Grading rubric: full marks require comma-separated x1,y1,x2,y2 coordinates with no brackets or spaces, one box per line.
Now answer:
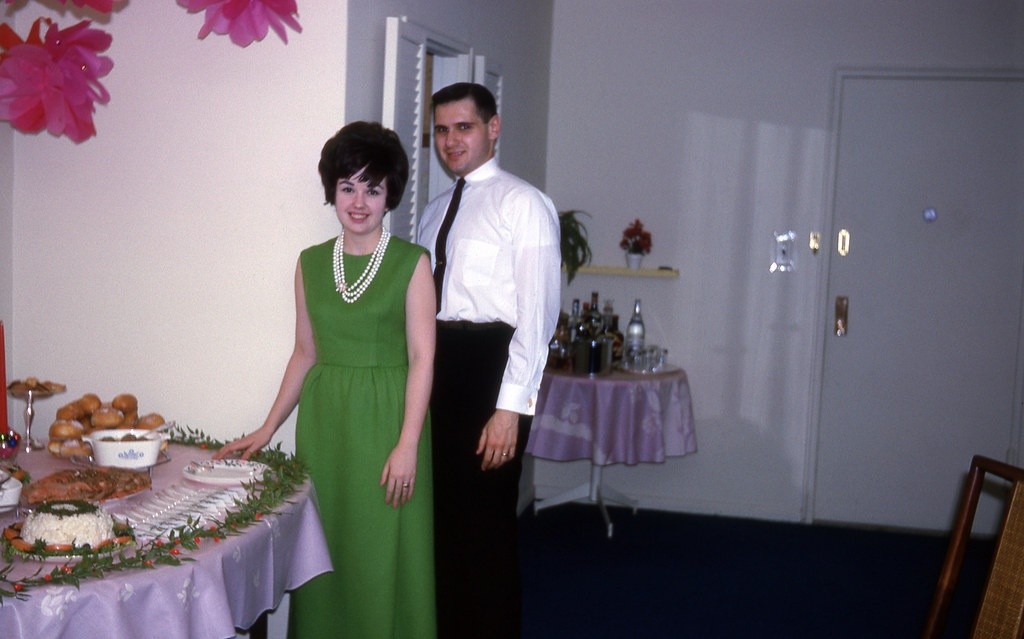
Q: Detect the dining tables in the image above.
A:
0,432,335,639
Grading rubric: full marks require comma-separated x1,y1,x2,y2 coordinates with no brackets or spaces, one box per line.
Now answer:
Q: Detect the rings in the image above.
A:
403,483,409,487
501,452,509,456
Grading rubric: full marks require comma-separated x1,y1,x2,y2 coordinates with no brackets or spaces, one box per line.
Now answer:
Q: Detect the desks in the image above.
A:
527,369,695,536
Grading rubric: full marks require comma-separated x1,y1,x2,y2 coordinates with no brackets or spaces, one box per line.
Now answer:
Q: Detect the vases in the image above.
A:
628,254,643,271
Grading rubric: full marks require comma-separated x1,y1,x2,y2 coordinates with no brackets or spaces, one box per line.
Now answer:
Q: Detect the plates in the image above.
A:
3,515,135,563
182,458,273,487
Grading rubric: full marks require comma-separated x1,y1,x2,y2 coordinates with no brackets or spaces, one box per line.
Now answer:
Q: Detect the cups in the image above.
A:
624,345,669,374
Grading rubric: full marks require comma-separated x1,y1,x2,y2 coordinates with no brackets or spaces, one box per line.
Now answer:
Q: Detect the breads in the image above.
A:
47,393,165,458
43,381,67,393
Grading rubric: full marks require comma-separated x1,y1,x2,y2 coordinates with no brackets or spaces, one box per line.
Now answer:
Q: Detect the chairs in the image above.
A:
922,454,1024,639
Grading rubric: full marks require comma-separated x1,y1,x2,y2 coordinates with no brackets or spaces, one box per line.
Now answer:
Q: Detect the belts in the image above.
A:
438,320,512,330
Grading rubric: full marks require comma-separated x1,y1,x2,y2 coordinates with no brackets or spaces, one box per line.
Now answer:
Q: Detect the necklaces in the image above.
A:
333,223,392,303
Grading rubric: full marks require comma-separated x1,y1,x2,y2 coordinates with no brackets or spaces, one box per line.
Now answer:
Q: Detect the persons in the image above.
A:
411,81,561,639
214,122,438,639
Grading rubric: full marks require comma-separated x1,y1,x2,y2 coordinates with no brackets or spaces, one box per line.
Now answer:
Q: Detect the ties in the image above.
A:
433,178,466,316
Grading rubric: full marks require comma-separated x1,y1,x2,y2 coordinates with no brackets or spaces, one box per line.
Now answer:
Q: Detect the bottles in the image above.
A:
548,292,645,380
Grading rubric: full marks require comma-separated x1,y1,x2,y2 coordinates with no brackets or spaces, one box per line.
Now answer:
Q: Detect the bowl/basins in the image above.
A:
0,472,23,512
83,428,171,468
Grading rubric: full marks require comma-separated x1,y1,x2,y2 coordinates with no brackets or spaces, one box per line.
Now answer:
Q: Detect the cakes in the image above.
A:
5,498,133,553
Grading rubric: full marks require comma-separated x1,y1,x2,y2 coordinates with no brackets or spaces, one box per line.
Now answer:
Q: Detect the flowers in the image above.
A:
176,0,303,46
0,0,117,140
619,218,653,254
